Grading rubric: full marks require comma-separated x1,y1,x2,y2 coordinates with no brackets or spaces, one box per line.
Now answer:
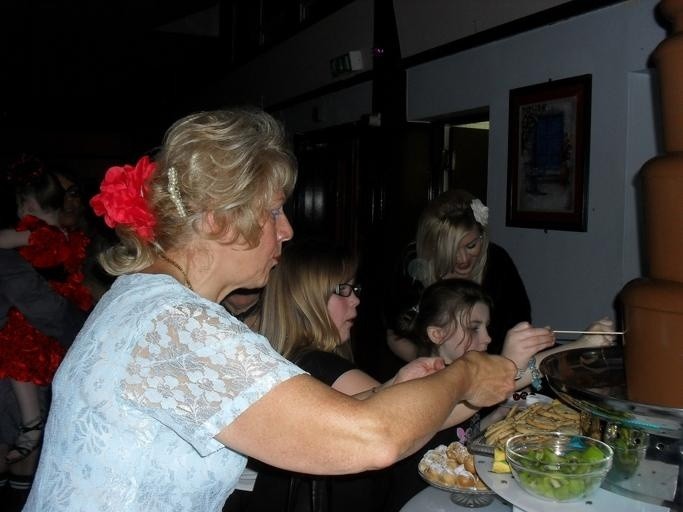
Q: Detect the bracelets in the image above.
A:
527,356,542,392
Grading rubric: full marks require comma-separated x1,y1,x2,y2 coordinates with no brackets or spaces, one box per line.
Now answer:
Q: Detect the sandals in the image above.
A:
4,418,45,466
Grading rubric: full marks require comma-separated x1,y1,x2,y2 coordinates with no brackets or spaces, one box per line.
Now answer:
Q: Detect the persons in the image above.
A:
48,162,106,308
414,278,617,491
386,188,532,443
253,243,555,512
19,104,521,512
0,244,90,511
0,172,91,464
217,288,289,511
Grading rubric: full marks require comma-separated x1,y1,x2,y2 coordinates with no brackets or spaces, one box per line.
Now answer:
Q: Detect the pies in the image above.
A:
484,398,581,448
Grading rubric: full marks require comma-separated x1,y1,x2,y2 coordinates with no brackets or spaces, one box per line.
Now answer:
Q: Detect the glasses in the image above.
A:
330,282,363,298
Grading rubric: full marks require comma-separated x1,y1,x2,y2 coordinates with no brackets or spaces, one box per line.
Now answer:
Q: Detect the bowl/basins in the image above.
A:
605,425,650,486
504,430,615,503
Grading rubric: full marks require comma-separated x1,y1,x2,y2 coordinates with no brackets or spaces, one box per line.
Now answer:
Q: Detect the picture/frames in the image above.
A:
503,73,592,232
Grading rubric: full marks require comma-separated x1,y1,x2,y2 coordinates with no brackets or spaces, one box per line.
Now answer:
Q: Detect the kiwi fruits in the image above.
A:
519,445,606,501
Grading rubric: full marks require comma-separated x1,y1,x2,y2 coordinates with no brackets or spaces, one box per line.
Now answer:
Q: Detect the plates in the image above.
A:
497,390,554,410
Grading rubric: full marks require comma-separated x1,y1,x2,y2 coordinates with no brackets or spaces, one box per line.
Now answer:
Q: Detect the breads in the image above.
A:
418,441,488,491
